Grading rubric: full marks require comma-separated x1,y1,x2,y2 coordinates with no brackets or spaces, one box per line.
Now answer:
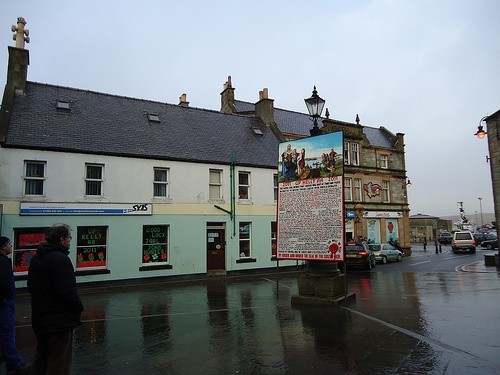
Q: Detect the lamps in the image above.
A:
406,180,412,187
474,116,488,139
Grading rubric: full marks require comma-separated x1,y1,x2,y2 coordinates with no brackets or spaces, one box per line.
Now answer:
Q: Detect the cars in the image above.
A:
368,243,404,264
345,241,376,270
439,229,498,254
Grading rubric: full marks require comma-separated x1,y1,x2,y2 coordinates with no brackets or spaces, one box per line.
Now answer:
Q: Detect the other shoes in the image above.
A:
6,363,31,375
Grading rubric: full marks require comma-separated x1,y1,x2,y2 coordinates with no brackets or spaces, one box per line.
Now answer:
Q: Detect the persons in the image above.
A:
27,222,85,375
0,236,34,375
347,235,427,254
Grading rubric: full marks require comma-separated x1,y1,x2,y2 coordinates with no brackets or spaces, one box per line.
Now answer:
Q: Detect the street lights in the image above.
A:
290,85,356,310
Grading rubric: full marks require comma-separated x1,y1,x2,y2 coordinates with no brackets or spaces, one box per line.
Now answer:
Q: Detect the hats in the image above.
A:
0,236,10,250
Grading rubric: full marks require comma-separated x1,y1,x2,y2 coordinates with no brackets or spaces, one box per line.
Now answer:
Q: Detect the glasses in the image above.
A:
64,236,72,241
